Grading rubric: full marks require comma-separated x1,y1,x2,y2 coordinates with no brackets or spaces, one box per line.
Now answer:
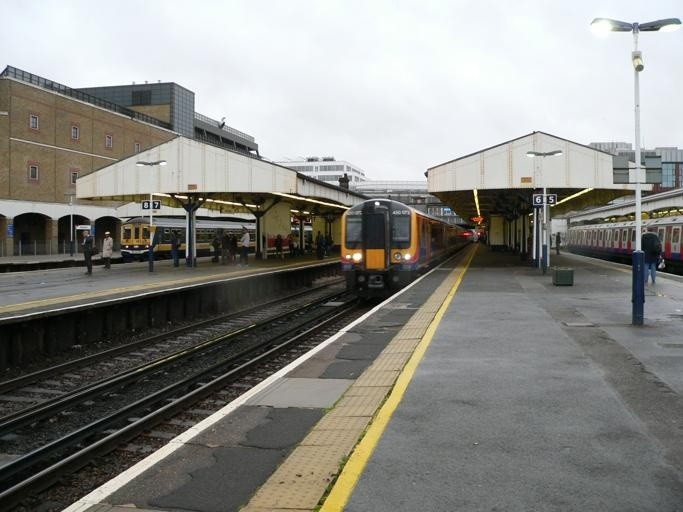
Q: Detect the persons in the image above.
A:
80,230,93,274
212,227,251,267
315,231,322,249
556,231,562,255
275,234,284,259
641,226,662,284
102,231,114,269
287,233,295,256
169,230,182,267
324,232,332,253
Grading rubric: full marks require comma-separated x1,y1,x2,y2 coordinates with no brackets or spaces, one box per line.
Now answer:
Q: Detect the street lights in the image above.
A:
589,17,683,326
526,150,564,276
136,160,167,272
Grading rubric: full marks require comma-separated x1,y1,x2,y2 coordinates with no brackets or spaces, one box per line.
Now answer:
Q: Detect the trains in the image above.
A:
566,188,683,276
120,217,313,263
340,198,473,301
